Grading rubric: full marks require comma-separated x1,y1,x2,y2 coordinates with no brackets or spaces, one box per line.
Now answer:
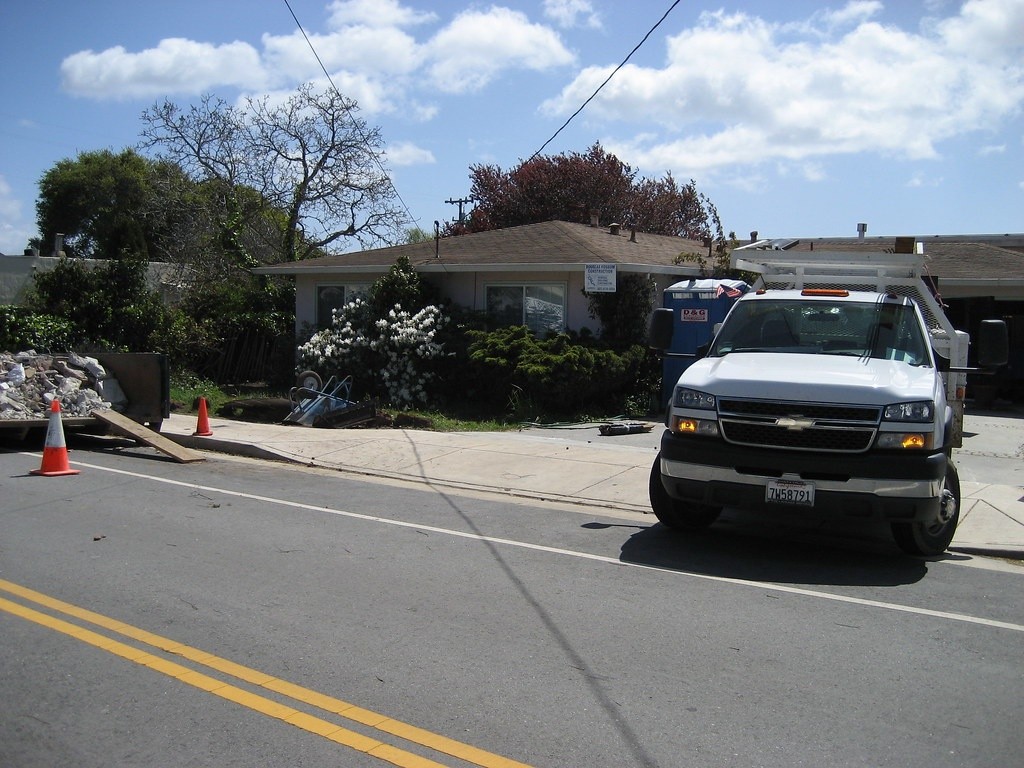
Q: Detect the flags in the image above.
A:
717,284,742,298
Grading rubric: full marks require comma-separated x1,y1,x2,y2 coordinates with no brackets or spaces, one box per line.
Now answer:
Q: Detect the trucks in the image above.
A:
648,238,1009,558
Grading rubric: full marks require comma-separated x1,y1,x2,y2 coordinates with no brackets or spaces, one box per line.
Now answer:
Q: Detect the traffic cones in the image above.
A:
29,399,81,477
191,396,213,436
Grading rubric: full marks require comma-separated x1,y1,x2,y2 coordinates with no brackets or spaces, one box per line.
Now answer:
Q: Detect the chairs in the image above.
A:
867,319,897,350
762,320,796,346
